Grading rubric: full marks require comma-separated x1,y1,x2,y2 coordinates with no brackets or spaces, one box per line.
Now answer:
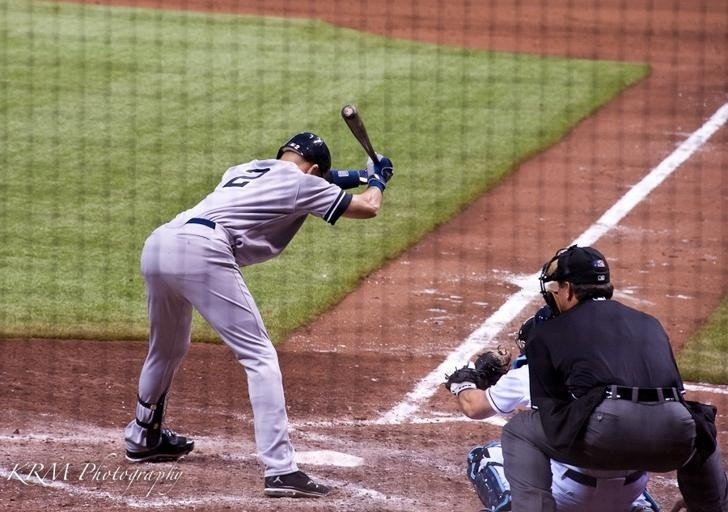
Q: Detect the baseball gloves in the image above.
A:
471,348,512,388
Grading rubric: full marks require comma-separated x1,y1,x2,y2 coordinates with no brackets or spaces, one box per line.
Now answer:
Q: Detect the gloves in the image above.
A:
366,152,394,193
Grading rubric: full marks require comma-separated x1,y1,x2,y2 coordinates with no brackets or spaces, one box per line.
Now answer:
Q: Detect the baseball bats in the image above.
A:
342,104,380,168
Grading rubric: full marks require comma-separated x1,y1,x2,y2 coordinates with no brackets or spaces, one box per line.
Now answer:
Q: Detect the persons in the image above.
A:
443,259,660,510
122,132,392,496
500,245,727,509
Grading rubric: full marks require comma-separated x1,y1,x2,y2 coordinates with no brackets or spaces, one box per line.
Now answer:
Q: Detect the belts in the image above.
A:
603,384,685,403
185,216,218,231
563,467,646,489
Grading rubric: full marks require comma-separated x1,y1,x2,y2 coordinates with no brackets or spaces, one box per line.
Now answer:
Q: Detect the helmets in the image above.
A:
538,243,611,316
276,131,332,176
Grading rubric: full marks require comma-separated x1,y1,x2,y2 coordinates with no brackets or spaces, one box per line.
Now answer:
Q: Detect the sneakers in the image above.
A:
125,431,196,463
262,469,331,498
